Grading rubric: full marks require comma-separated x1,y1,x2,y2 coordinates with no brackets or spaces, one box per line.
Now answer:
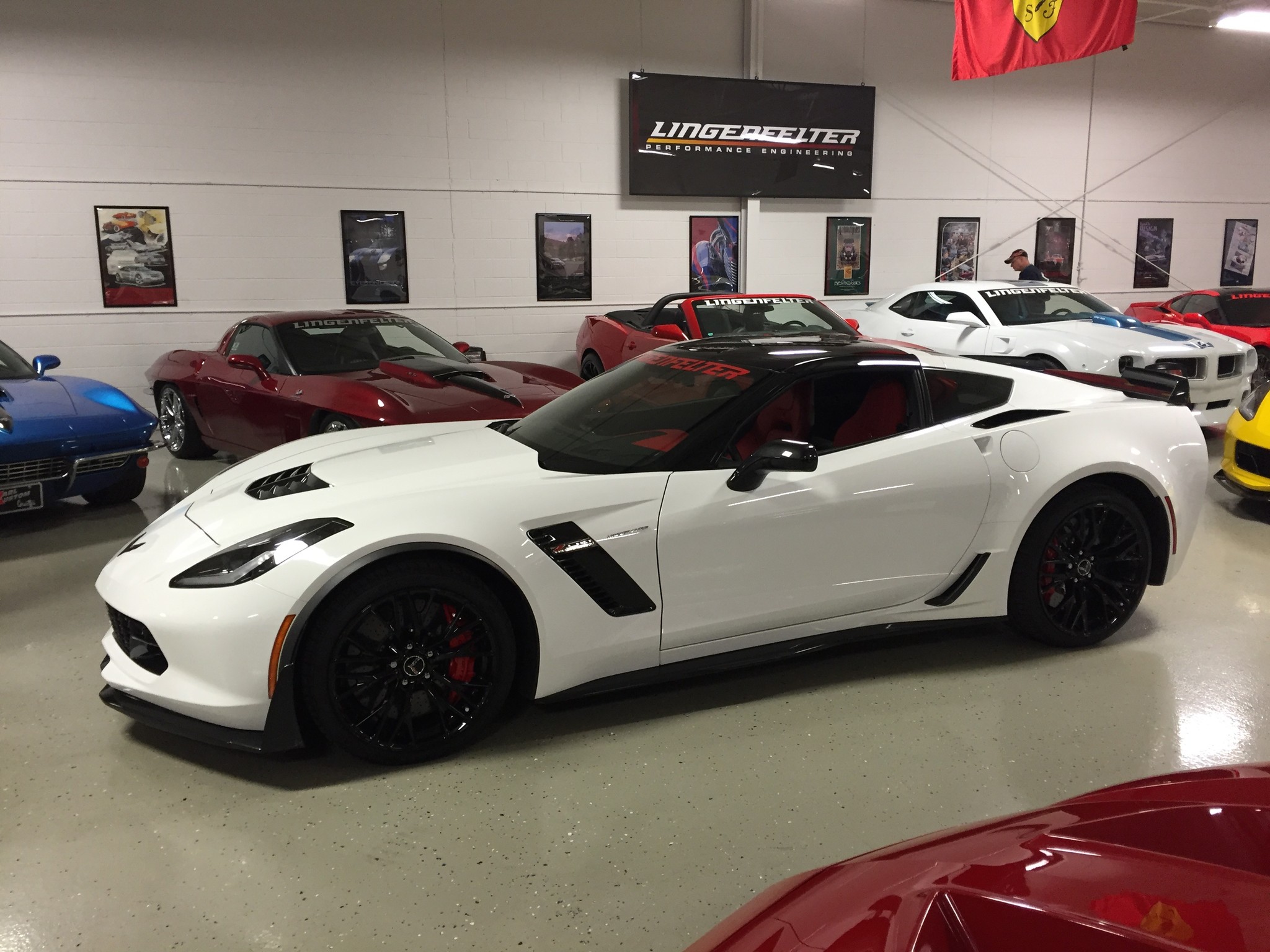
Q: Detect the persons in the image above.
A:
1004,249,1053,314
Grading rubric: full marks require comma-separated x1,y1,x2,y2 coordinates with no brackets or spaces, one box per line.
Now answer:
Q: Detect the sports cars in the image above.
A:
104,240,169,288
576,291,935,383
685,763,1270,951
841,278,1269,500
92,334,1211,766
0,339,167,518
547,257,566,269
145,308,613,462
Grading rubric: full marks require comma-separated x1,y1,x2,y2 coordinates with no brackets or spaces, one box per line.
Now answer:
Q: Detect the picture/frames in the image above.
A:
1220,219,1259,287
93,205,178,308
689,215,739,293
535,212,592,302
824,216,872,296
1133,218,1174,288
1034,217,1076,284
340,209,410,305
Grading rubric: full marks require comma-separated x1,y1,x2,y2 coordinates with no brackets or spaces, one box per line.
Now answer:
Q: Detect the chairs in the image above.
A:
289,328,328,372
331,323,398,365
832,378,906,450
736,381,814,458
742,304,766,332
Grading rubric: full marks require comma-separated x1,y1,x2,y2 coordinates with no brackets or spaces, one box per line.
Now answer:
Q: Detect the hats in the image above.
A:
1004,249,1028,264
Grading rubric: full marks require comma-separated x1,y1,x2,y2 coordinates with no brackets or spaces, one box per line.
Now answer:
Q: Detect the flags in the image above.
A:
950,1,1139,81
